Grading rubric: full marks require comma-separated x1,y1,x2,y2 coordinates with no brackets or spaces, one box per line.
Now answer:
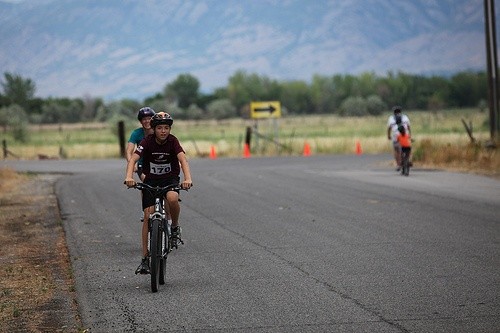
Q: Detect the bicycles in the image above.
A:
124,170,193,292
400,150,410,175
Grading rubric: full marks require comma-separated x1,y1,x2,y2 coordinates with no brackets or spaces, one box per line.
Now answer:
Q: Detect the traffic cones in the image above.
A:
303,142,311,157
356,142,362,155
209,145,217,159
243,143,252,158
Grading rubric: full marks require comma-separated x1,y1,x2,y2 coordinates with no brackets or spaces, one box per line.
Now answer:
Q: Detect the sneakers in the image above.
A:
141,257,151,274
169,224,182,246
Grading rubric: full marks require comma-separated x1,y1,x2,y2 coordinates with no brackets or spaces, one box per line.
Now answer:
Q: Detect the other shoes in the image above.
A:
140,212,144,220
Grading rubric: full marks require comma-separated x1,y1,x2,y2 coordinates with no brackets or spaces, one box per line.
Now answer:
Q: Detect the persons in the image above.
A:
126,107,157,221
388,107,412,176
125,112,193,274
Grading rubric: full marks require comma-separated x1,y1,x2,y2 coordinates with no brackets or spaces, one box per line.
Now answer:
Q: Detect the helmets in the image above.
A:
149,111,174,127
138,107,156,120
